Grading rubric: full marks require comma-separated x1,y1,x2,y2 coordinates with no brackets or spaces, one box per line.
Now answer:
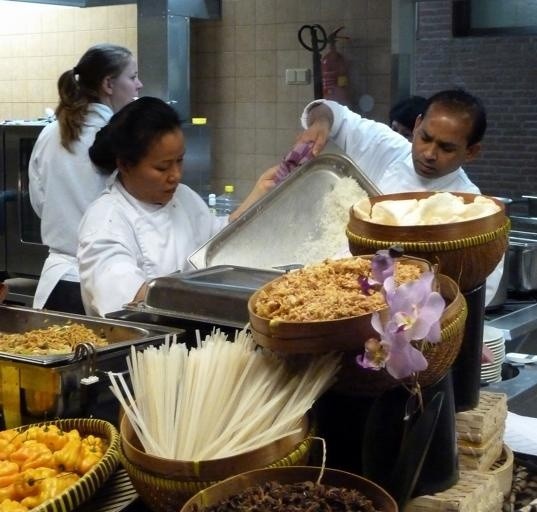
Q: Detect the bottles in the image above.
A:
209,194,217,216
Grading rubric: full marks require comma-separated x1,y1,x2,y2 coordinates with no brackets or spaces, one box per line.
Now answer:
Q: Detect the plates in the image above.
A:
480,324,507,385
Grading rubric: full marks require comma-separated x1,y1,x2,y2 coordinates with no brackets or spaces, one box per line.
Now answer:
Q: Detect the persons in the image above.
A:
77,96,280,320
293,90,505,306
389,95,427,142
28,43,144,315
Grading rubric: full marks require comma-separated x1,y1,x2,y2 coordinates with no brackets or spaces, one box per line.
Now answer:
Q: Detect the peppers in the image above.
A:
0,425,103,511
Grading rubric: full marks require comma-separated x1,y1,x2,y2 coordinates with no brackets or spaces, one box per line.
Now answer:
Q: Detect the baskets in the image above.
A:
406,273,470,390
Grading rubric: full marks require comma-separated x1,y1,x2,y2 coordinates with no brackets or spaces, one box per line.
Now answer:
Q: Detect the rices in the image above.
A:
299,175,371,266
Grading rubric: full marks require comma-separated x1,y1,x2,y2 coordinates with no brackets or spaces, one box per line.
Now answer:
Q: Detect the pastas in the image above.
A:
107,321,344,461
